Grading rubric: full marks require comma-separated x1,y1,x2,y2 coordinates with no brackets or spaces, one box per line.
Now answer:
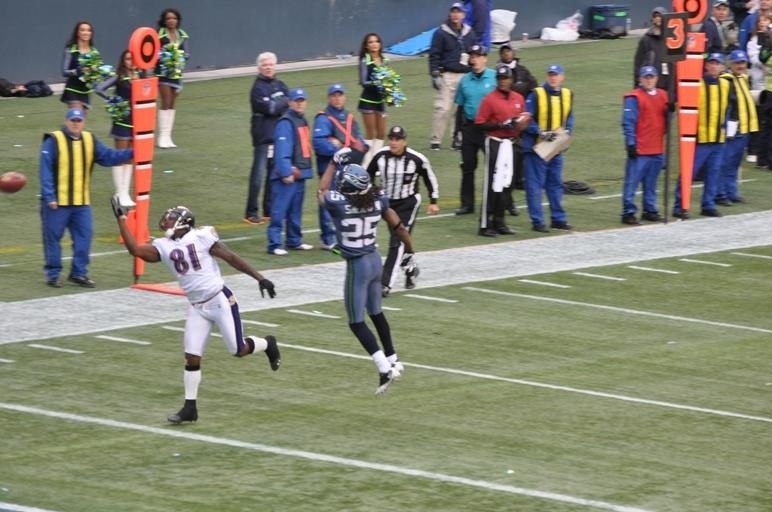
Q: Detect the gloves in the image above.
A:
627,146,636,159
451,131,463,147
332,147,352,165
431,71,442,90
400,253,416,273
110,196,130,219
540,130,557,142
498,118,516,129
256,279,276,299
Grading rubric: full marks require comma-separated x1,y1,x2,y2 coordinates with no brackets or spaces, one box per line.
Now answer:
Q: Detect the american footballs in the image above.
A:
0,172,29,193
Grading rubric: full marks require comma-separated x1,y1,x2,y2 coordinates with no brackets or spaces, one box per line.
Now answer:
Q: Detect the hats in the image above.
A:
547,65,564,75
712,0,729,8
639,65,657,77
327,84,345,94
387,125,408,139
499,43,512,52
494,65,513,78
652,6,668,15
467,44,488,56
65,109,84,120
449,3,464,11
288,89,306,100
729,50,748,64
706,52,726,64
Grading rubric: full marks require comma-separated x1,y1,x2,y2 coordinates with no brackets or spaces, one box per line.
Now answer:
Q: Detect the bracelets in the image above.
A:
393,220,402,231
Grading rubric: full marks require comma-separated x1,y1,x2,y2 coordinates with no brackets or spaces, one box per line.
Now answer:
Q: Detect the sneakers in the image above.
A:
67,274,96,288
496,226,516,235
243,216,266,225
622,215,638,225
641,212,663,221
406,267,420,288
673,210,689,219
290,243,315,250
375,365,400,396
320,242,335,250
478,228,496,237
508,203,520,216
701,208,722,217
381,286,390,297
264,335,281,371
391,359,404,373
532,225,549,233
551,223,573,230
716,198,733,206
428,144,440,151
733,197,745,204
755,160,768,169
262,216,271,221
450,145,461,152
268,248,289,256
168,408,199,424
48,280,63,288
515,177,526,189
455,206,474,215
746,155,757,163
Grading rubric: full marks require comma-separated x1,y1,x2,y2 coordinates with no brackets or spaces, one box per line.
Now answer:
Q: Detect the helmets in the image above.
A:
335,163,370,194
159,206,194,230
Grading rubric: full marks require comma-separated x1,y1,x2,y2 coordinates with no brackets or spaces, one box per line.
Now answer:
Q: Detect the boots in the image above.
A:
155,109,169,149
168,109,176,148
362,139,373,169
124,164,136,206
110,165,129,207
373,138,384,157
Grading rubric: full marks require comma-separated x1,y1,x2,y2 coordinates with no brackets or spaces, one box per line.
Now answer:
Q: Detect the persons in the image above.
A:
38,107,133,288
474,65,515,237
311,83,364,251
495,44,537,190
155,8,191,147
522,63,573,233
429,2,478,152
621,65,671,226
453,44,519,217
465,0,492,53
357,33,395,141
633,7,676,109
243,52,289,225
317,147,404,397
110,195,280,425
267,88,313,256
59,21,102,114
367,125,440,298
94,50,140,209
672,0,771,219
